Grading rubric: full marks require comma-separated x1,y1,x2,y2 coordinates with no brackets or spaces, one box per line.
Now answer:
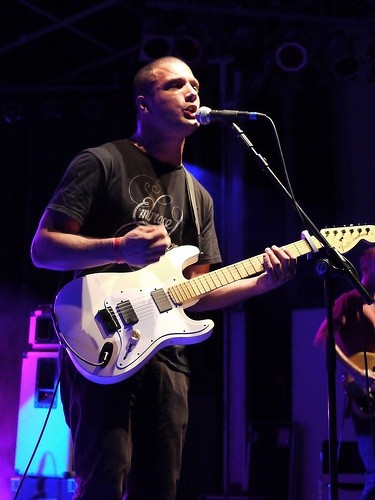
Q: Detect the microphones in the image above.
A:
196,106,266,125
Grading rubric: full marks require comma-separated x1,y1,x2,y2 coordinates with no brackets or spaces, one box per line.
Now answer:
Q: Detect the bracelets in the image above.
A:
113,237,120,264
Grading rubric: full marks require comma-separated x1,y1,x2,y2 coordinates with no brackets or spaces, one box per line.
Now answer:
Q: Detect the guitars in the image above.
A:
343,350,375,422
52,222,375,388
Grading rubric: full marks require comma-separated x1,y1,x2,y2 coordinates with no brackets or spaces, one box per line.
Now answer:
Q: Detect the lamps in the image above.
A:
4,98,102,123
327,25,360,79
140,12,208,65
276,26,309,72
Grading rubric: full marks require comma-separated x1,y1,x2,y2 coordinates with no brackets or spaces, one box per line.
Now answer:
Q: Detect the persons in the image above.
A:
31,57,293,500
